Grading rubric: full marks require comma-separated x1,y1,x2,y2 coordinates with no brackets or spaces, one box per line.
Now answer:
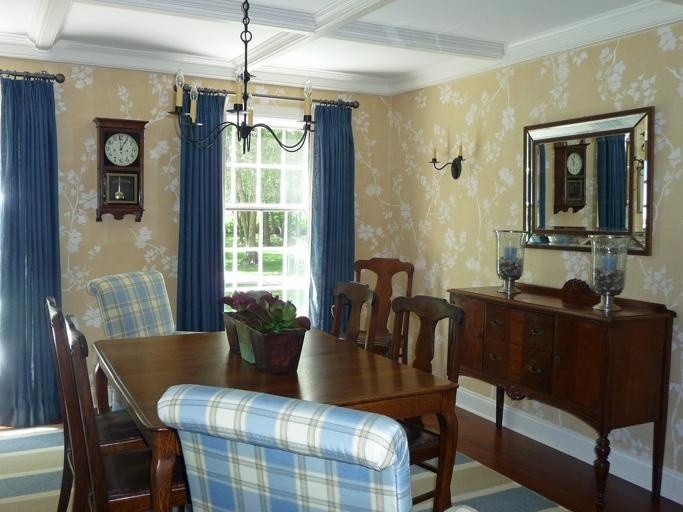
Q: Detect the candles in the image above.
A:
502,246,518,260
601,254,616,271
458,145,462,157
432,148,436,157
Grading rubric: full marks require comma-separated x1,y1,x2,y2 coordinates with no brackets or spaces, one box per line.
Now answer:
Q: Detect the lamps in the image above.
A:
167,0,315,158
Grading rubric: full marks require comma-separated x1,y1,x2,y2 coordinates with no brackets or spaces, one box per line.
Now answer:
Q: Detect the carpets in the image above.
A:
0,423,570,512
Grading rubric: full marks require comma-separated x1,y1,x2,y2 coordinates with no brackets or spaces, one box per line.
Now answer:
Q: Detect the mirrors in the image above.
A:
518,106,654,256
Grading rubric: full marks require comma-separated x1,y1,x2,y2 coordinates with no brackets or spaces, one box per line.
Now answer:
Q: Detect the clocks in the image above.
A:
93,114,148,224
552,142,587,214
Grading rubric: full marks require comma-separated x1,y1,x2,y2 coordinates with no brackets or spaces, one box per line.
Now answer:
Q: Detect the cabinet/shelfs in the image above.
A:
444,285,677,511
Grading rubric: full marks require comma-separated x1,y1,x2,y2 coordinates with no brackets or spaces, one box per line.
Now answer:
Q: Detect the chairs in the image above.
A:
43,258,464,512
